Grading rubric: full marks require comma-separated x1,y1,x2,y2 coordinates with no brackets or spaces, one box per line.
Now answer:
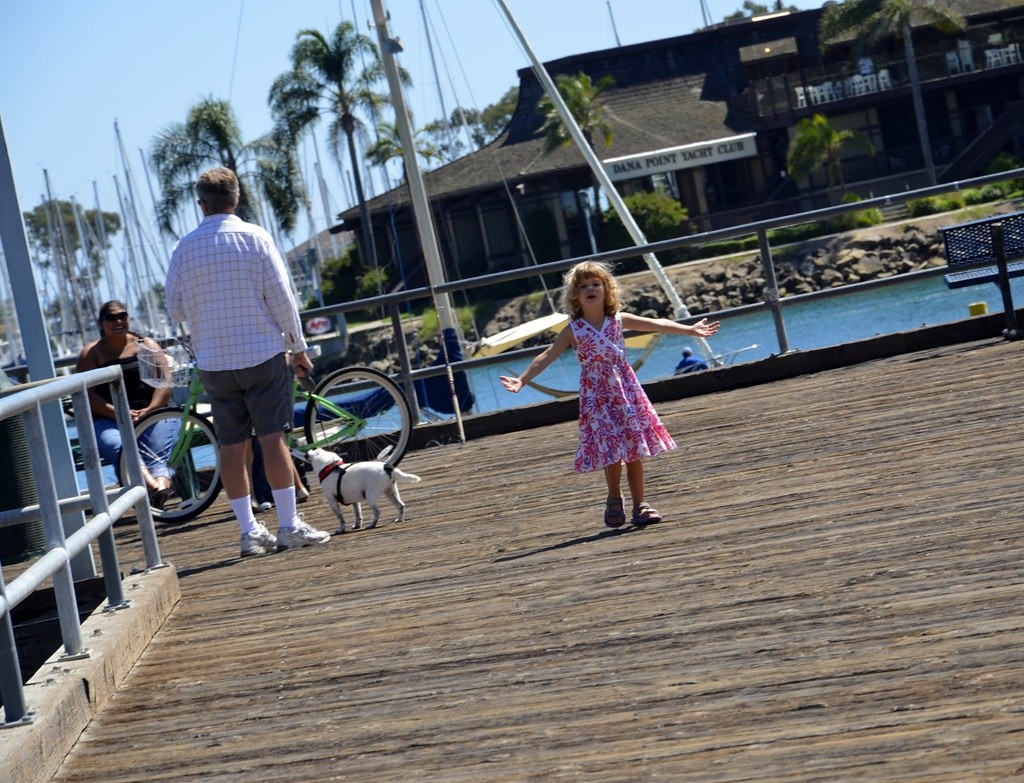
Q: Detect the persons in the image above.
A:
163,166,331,560
675,347,708,375
499,261,721,529
72,299,176,506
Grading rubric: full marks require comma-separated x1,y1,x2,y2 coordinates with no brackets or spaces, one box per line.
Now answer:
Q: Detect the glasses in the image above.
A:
102,311,128,322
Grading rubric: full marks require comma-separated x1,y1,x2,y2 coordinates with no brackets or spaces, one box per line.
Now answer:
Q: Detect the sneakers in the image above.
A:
240,520,278,557
277,512,331,548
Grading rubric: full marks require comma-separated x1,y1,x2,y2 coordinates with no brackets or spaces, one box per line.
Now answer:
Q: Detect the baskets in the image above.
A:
135,336,192,388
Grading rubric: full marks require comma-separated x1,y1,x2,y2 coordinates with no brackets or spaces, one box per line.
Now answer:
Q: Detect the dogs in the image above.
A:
308,447,422,534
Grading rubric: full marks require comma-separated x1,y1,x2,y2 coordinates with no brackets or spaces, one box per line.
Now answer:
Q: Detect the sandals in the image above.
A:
630,502,661,524
605,497,625,527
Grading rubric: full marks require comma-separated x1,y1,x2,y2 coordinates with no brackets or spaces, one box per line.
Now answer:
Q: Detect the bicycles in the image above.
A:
116,319,414,526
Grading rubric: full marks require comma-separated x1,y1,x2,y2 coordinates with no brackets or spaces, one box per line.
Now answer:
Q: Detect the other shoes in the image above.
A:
251,500,265,513
150,488,175,509
295,487,309,504
